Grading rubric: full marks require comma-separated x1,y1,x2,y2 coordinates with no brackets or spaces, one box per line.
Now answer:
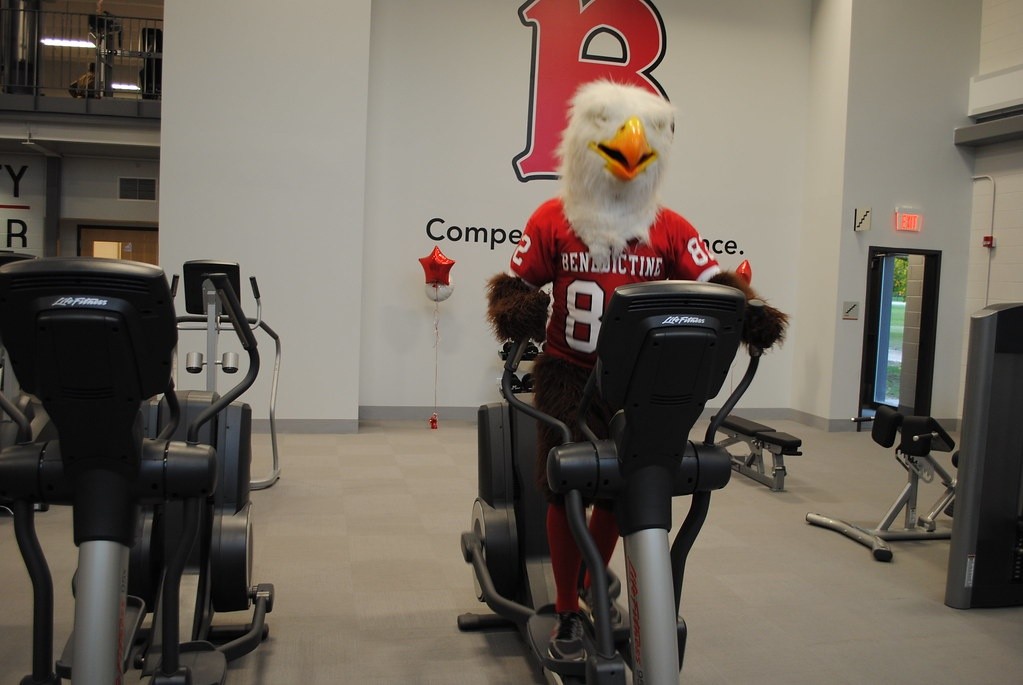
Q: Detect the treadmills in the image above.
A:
456,276,765,684
0,262,282,685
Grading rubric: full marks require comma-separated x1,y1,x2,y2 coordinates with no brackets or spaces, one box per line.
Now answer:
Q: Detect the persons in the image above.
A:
485,78,789,663
68,62,104,98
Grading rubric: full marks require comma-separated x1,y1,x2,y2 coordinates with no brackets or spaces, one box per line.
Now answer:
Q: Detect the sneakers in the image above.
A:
548,609,587,661
578,585,624,628
